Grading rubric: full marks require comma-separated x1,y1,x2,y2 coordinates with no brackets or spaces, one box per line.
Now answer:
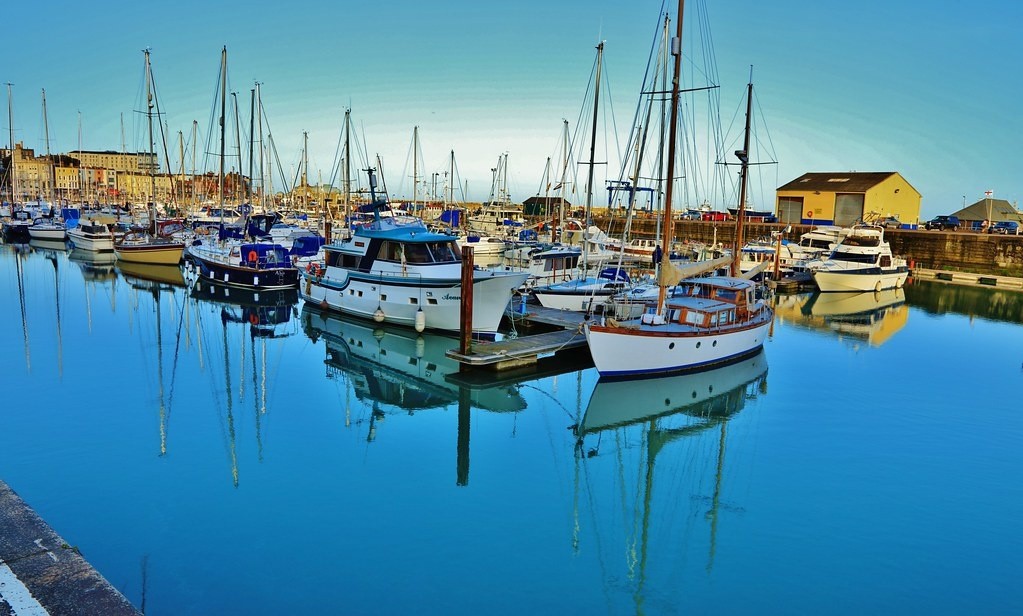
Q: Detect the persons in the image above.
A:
438,243,450,261
394,244,407,260
981,219,988,232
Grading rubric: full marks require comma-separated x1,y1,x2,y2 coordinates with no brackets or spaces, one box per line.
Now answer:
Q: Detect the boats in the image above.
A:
300,302,529,443
801,288,906,355
810,224,909,292
727,207,772,216
300,166,532,342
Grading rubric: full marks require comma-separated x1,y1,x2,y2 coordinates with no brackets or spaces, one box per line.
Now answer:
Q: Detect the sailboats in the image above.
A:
115,260,185,456
571,347,769,616
2,236,33,370
582,0,774,379
68,248,117,334
30,239,69,381
183,276,298,488
0,0,844,318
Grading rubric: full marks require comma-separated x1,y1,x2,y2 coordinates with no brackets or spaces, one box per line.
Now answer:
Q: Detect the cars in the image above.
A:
680,210,701,220
872,216,901,228
988,221,1019,235
702,210,729,221
925,215,961,231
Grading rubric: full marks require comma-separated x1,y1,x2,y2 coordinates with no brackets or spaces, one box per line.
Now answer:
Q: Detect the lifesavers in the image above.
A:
306,263,321,276
630,270,639,278
248,251,257,261
808,211,812,217
